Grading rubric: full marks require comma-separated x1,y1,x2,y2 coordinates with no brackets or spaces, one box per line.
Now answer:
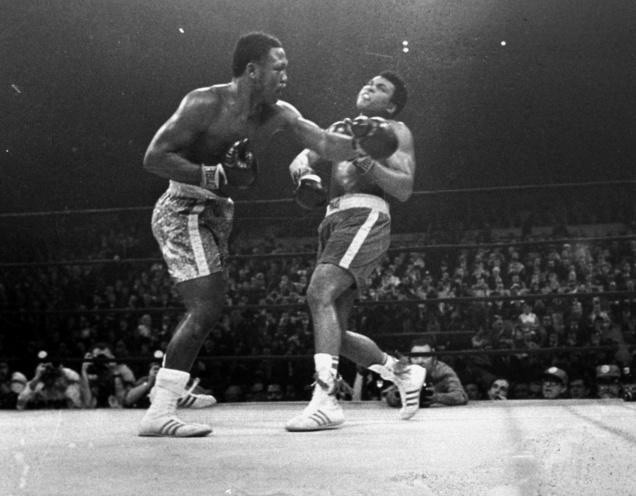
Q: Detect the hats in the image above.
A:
543,366,568,385
593,364,622,382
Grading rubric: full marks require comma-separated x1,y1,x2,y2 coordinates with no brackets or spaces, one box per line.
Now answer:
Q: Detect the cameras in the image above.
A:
86,354,111,378
40,367,61,386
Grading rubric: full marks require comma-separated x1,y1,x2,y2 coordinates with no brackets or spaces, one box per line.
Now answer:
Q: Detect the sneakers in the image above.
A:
396,363,428,420
148,382,217,409
285,402,345,431
139,411,213,437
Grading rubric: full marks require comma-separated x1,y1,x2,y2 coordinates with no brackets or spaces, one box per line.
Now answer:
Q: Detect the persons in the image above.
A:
282,70,430,433
1,202,636,414
131,29,399,436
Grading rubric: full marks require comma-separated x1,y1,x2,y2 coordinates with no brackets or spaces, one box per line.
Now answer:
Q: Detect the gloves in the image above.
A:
351,113,398,161
329,117,375,175
212,136,257,196
290,165,327,211
